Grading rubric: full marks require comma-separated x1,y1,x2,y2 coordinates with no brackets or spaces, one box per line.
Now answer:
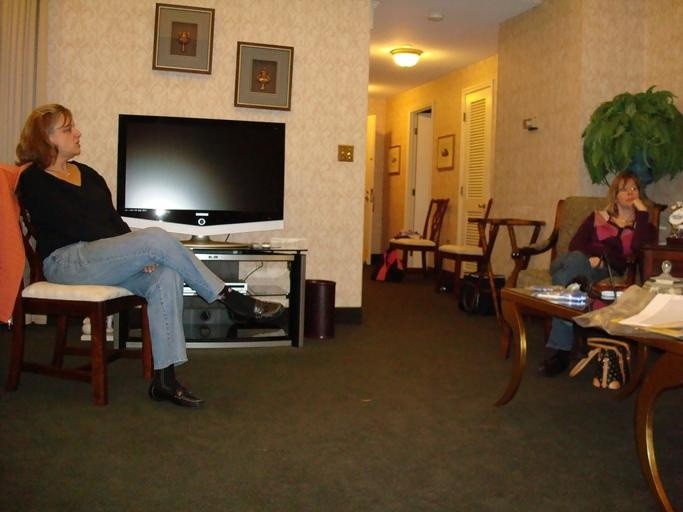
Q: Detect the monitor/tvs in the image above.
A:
117,113,284,250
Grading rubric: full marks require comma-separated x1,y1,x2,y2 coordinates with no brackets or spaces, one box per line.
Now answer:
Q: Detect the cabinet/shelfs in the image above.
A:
467,217,546,329
113,247,307,350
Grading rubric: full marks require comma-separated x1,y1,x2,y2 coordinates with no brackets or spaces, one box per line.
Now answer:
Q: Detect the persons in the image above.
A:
536,172,654,377
13,104,284,409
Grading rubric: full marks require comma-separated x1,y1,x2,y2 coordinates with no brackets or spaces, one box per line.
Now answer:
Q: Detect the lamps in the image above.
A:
389,47,424,68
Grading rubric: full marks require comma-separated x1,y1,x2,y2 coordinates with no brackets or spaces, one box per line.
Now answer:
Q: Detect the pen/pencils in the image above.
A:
603,252,617,299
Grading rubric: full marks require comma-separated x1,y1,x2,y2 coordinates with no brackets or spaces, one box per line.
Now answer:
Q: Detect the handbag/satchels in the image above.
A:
569,338,631,392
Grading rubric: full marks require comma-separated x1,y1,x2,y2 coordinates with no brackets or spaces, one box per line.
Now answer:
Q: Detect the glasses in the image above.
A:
617,186,637,192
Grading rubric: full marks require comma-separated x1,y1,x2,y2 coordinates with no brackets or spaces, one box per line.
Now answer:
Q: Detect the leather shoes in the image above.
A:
150,381,205,407
537,355,569,377
229,299,285,325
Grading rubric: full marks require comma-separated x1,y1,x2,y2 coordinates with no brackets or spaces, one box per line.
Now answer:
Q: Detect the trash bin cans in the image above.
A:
305,279,336,339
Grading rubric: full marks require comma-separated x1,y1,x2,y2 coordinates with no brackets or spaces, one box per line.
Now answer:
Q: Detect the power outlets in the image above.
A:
338,144,355,163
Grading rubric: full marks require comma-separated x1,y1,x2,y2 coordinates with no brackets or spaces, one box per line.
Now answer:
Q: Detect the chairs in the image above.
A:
388,197,449,278
0,161,154,407
436,197,495,299
507,195,666,348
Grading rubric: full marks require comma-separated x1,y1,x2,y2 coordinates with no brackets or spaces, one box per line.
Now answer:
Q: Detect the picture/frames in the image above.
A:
151,2,216,76
387,145,401,175
233,40,295,111
436,133,455,172
